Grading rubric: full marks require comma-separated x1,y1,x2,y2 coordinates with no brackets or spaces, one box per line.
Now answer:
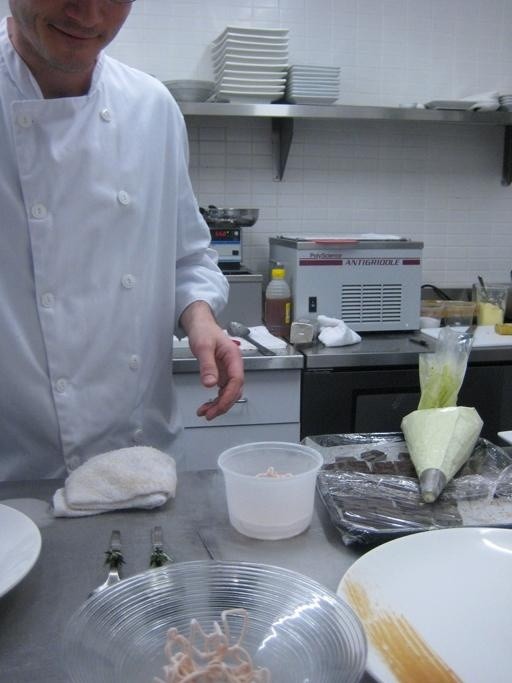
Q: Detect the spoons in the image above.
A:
227,321,275,357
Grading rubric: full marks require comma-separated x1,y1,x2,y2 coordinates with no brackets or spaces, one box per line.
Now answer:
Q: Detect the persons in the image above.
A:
0,0,246,480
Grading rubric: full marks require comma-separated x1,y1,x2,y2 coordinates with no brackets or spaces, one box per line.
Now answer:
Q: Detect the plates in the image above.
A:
420,322,512,347
335,527,512,683
212,22,340,106
1,502,45,604
423,92,512,114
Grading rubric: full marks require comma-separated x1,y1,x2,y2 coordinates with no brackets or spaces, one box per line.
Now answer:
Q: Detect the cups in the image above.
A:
420,281,510,326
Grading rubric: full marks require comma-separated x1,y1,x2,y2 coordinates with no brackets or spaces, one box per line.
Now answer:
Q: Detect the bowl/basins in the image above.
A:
157,78,215,103
60,560,369,683
217,440,323,540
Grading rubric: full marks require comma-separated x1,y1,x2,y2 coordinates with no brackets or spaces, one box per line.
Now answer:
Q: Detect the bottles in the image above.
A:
266,269,291,340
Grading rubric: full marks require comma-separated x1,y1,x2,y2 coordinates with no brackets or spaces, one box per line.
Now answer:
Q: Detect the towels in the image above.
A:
51,447,181,515
317,315,364,347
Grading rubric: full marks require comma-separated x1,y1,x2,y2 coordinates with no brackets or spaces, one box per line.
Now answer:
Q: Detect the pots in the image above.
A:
197,205,259,227
